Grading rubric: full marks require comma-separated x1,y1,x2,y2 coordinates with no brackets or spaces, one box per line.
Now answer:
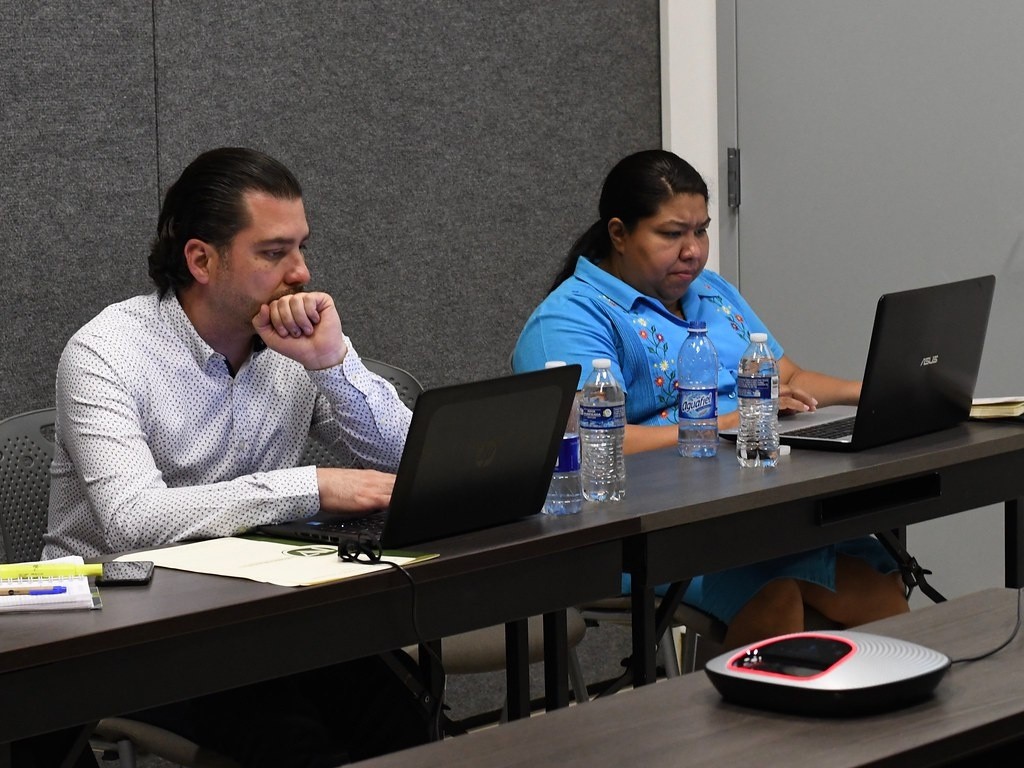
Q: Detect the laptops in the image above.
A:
256,363,583,550
718,274,996,452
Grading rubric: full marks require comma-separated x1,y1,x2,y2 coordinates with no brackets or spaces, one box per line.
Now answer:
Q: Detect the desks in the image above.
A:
0,403,1024,768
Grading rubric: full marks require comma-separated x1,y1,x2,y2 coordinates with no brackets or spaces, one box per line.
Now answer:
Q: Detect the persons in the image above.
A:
509,148,911,654
39,148,435,768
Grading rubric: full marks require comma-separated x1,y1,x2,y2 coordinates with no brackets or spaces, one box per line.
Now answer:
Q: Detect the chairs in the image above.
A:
508,348,730,696
0,406,236,768
354,356,589,724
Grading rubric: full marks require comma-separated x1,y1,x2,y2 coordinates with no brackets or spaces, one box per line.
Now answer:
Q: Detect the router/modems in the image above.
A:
704,630,950,718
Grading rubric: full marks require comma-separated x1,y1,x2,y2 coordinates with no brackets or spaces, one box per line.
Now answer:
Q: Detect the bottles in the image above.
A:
677,321,718,458
737,332,780,468
542,361,583,514
579,358,626,503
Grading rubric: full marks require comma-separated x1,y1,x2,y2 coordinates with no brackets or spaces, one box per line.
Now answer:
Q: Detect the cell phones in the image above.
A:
95,560,154,586
